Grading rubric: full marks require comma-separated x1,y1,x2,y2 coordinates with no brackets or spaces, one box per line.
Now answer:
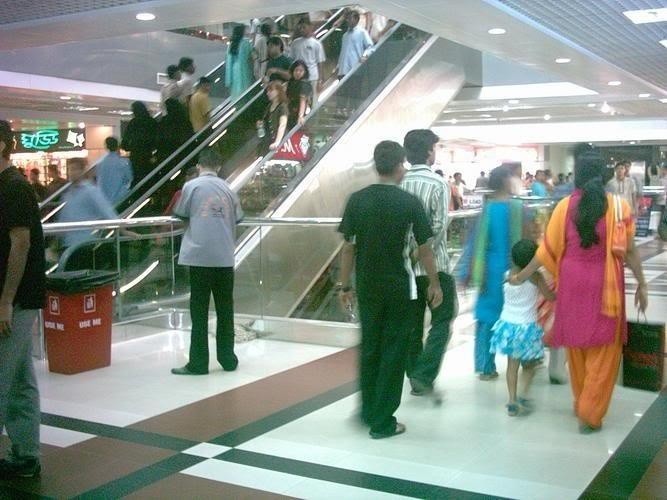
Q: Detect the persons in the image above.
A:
337,140,443,439
508,151,648,434
537,263,569,384
30,169,45,202
623,160,642,197
47,164,67,195
337,11,374,83
523,169,576,198
0,120,49,478
95,137,134,206
158,98,200,189
459,165,540,380
176,57,196,93
290,19,325,107
120,101,166,189
475,171,490,187
254,23,271,82
189,77,212,132
256,81,288,156
58,158,141,274
606,163,638,218
453,173,466,196
171,149,245,375
264,38,293,83
435,170,462,211
489,239,556,417
224,25,253,95
288,61,313,126
399,129,459,396
160,65,181,111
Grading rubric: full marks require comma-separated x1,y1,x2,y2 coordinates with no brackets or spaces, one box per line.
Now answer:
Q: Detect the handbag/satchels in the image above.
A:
611,194,628,256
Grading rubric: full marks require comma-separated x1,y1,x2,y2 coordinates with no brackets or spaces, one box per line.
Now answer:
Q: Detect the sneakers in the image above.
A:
508,404,518,416
0,458,40,478
172,365,208,375
518,398,535,412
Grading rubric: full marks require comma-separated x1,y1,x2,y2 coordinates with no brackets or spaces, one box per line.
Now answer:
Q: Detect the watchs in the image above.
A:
339,286,353,293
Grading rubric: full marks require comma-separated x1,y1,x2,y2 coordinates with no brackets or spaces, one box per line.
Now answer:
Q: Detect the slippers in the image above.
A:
369,422,406,437
480,370,498,381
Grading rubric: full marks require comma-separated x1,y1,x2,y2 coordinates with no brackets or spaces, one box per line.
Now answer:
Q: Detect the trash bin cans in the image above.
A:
42,269,120,375
